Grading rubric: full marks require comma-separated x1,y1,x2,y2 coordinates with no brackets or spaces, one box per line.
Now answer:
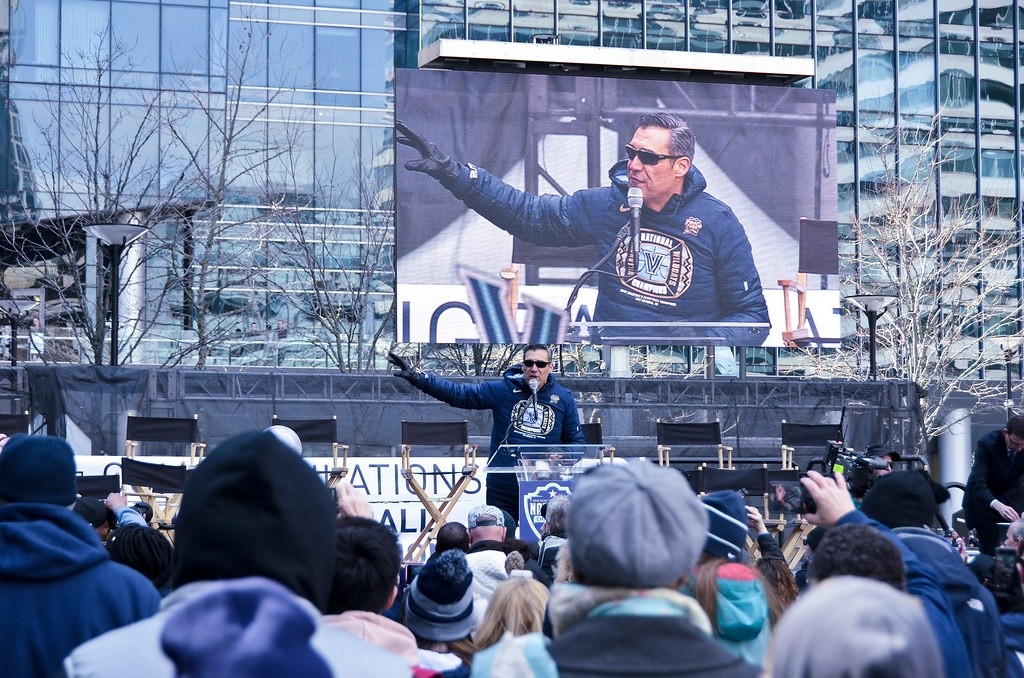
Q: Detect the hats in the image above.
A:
465,549,524,600
161,577,333,678
500,509,516,530
168,431,335,606
74,497,107,527
917,468,950,504
567,463,709,587
863,471,936,527
135,503,153,523
403,548,474,640
0,434,77,505
700,490,749,561
863,444,901,462
943,531,953,537
467,504,505,529
546,495,569,533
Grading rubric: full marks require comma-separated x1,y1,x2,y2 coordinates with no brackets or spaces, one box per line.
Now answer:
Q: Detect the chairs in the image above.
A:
0,411,844,569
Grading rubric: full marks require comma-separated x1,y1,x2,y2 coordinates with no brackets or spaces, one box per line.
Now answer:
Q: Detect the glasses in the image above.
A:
625,144,684,165
523,360,551,368
1008,434,1024,448
888,462,894,468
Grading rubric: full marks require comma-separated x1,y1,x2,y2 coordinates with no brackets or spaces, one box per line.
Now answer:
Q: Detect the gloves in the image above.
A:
849,468,875,497
389,353,420,383
395,119,456,180
664,319,708,337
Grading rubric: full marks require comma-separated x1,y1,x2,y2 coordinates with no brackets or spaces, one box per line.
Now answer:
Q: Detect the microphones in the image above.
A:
627,188,643,274
529,379,539,420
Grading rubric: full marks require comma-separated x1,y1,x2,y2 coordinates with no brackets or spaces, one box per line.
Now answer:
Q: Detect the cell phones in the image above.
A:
993,547,1021,598
406,564,424,587
768,481,817,514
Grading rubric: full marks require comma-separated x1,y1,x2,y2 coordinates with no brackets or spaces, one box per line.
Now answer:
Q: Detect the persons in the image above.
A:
388,345,586,527
395,113,772,344
0,417,1024,678
